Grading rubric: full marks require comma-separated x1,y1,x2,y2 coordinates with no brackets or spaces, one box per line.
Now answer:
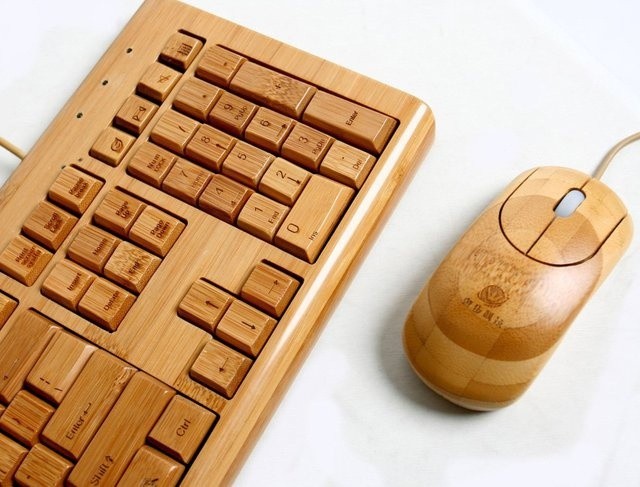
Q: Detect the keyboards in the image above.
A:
0,0,437,487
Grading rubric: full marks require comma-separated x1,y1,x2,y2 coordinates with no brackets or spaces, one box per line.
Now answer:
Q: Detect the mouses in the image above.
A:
405,166,635,414
